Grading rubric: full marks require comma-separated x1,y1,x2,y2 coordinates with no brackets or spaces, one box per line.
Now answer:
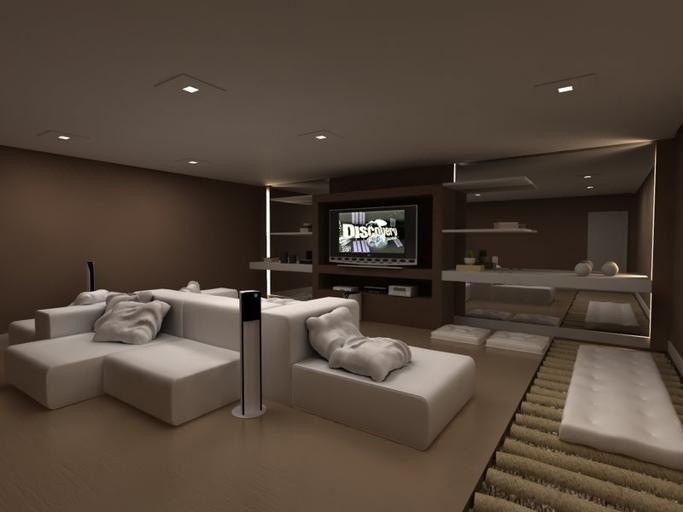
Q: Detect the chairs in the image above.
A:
6,287,478,452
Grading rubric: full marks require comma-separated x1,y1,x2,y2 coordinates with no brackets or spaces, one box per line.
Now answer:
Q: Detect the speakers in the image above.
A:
85,260,96,291
231,290,267,418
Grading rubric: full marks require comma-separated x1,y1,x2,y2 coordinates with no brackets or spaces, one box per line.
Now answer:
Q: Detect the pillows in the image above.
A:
67,289,108,306
302,304,368,360
92,290,154,333
329,336,416,382
92,299,172,344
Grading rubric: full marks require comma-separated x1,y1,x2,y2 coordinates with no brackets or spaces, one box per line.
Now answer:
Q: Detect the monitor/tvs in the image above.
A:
329,206,417,268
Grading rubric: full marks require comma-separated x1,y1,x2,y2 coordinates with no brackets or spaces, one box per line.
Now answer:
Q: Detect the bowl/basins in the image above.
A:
464,257,476,265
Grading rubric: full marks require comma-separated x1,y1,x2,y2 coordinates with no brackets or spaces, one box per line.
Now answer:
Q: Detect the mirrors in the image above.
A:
464,283,649,338
